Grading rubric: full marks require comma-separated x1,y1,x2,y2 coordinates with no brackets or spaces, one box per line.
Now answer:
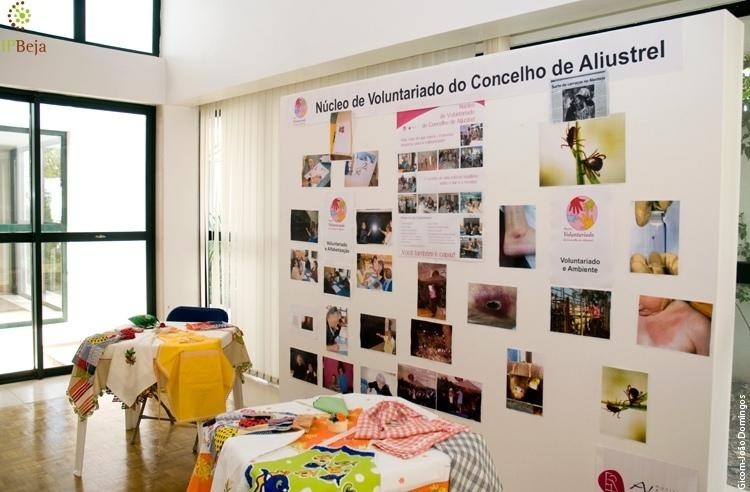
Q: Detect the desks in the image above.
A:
65,321,253,479
184,394,505,492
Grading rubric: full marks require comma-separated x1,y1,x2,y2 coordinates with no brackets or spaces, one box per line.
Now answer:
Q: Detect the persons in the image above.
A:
504,205,536,257
636,295,709,356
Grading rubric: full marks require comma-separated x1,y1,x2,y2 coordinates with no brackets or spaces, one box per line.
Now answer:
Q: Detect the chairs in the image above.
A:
131,306,229,455
